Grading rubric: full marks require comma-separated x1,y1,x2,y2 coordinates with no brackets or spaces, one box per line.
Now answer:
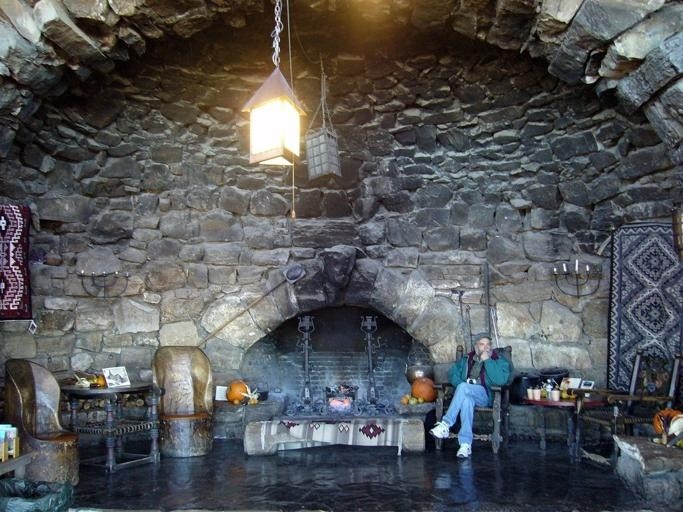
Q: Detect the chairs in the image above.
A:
151,344,218,458
564,349,678,470
5,357,81,488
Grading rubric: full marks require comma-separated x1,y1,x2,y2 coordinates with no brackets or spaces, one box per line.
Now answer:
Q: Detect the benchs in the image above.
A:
241,420,427,457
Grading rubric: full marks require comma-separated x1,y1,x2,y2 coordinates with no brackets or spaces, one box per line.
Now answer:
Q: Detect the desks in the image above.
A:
58,380,160,473
0,447,32,482
514,397,605,455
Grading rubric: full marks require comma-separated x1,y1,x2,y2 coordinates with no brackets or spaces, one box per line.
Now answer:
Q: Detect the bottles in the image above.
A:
0,423,18,461
545,379,552,399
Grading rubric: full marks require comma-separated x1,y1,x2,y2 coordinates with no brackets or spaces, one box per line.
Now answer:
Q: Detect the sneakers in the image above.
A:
429,422,449,438
456,443,472,458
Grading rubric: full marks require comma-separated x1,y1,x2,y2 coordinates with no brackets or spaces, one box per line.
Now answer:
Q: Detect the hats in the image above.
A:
474,333,492,344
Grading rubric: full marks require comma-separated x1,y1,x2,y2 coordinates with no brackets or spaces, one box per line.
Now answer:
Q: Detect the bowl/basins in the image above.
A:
254,391,268,401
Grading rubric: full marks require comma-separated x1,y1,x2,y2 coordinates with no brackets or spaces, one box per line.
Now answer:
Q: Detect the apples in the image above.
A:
248,399,258,406
233,399,240,405
562,390,577,400
677,440,683,448
541,389,548,398
401,394,425,406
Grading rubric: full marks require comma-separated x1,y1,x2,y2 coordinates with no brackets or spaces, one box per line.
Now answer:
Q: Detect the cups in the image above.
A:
551,390,559,401
526,388,533,400
532,389,540,400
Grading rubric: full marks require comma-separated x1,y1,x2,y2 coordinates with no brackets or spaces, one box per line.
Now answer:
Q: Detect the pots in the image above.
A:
509,370,540,406
536,365,569,389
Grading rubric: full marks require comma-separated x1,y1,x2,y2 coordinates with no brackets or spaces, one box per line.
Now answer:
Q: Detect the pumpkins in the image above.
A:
227,381,246,401
412,378,436,400
652,407,683,434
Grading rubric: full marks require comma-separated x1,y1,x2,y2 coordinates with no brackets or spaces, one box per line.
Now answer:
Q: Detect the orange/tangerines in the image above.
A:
96,375,107,387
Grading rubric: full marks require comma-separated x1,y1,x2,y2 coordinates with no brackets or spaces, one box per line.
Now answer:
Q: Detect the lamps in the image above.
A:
239,0,304,175
550,257,602,298
